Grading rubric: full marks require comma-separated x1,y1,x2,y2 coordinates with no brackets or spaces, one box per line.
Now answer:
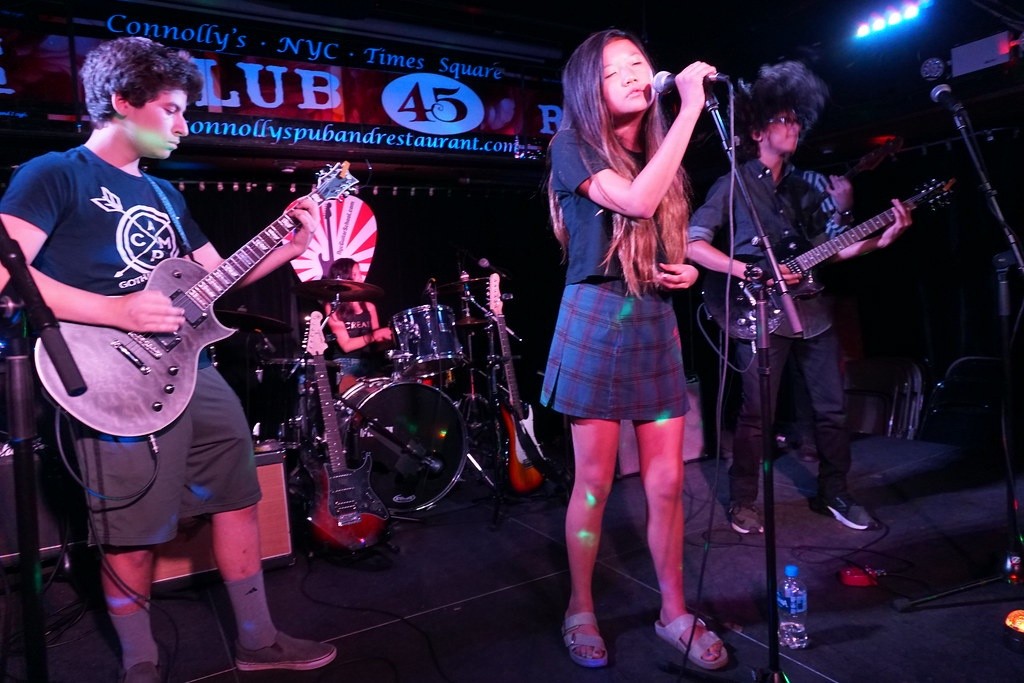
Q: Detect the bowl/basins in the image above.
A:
1003,608,1024,655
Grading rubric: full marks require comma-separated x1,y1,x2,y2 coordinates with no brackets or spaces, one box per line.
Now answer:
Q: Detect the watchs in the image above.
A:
838,210,852,215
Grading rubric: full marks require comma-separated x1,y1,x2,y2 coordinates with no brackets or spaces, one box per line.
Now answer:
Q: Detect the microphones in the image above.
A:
261,334,276,353
422,278,433,298
424,456,446,474
931,84,967,115
479,258,507,279
652,70,730,94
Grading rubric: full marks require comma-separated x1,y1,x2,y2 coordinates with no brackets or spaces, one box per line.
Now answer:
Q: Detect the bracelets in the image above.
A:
363,333,375,344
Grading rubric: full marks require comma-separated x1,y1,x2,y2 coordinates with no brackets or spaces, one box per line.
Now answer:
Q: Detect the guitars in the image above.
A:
486,273,548,497
34,161,360,438
301,311,392,566
797,136,904,220
702,179,961,341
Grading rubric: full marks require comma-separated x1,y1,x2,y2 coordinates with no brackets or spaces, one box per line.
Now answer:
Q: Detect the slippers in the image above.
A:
561,612,609,667
655,616,728,669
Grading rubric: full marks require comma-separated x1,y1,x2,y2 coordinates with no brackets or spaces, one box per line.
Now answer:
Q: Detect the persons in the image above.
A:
540,30,730,671
326,258,396,394
685,63,911,532
0,38,336,683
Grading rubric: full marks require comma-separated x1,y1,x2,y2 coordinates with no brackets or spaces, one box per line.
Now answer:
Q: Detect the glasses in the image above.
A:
762,115,801,128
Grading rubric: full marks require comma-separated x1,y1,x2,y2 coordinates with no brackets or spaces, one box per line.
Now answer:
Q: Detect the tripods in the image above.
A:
457,325,498,456
894,113,1024,617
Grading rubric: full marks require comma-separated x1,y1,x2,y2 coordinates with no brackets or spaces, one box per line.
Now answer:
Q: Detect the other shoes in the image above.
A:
235,628,337,671
118,657,159,683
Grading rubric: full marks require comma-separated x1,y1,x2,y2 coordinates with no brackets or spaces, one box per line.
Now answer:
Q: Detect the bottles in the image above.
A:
777,565,811,651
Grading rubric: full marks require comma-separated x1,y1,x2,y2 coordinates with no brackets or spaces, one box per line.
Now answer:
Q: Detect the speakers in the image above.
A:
0,439,94,567
150,440,295,593
615,373,709,478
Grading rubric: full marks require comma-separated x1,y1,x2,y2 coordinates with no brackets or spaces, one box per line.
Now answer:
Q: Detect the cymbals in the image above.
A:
455,316,489,329
437,277,489,294
290,278,385,303
213,307,295,334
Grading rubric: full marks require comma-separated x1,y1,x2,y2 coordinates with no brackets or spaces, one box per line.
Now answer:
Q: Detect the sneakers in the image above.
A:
729,499,764,535
821,492,877,529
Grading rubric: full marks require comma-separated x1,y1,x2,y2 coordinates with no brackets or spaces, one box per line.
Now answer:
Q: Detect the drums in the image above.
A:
256,358,341,451
313,376,469,514
453,393,498,484
389,304,465,378
